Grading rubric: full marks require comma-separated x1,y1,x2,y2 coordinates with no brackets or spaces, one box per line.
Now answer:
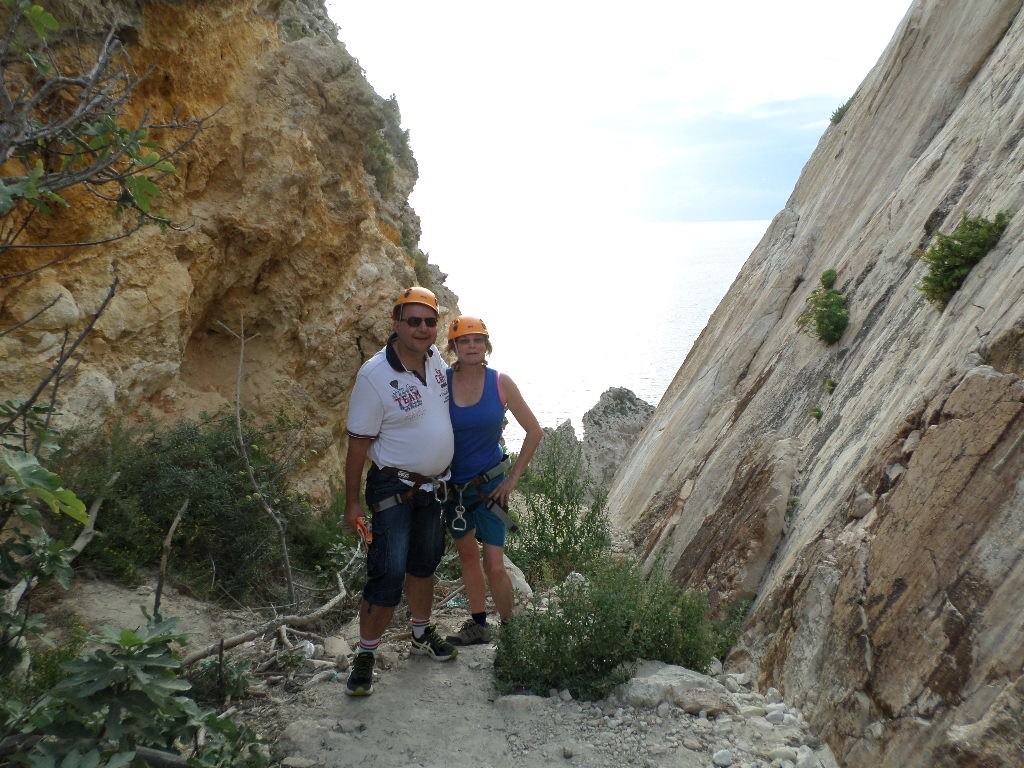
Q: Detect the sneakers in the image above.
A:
445,619,491,645
410,625,458,662
345,652,377,695
494,619,514,637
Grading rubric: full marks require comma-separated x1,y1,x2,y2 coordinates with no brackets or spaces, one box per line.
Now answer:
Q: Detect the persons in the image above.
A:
343,287,458,696
441,315,543,648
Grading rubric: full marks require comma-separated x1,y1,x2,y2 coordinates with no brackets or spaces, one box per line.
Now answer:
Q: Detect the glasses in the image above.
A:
398,317,438,328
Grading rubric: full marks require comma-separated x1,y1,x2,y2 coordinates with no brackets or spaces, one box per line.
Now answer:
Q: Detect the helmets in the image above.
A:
448,316,489,343
392,287,439,320
